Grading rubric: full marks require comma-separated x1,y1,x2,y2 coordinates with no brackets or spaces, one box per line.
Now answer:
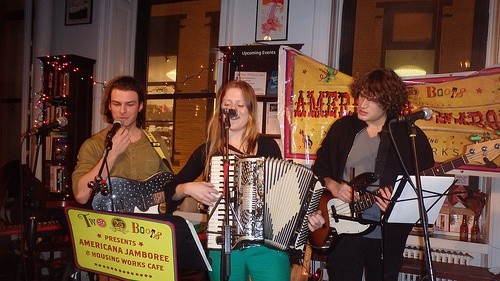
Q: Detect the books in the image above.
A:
234,71,278,95
48,72,69,96
46,104,67,125
45,131,68,160
257,102,281,148
50,165,65,193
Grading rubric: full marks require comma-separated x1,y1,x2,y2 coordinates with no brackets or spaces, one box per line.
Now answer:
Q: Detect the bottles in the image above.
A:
460,214,467,241
471,216,479,243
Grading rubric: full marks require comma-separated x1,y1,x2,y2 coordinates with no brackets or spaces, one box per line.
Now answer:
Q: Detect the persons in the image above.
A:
311,69,435,281
164,80,325,281
72,76,174,281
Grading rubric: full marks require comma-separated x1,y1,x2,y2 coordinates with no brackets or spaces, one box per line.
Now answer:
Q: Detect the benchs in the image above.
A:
311,248,500,281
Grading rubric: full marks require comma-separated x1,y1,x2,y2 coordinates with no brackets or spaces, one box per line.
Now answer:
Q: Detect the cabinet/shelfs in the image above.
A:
218,44,304,163
36,54,97,201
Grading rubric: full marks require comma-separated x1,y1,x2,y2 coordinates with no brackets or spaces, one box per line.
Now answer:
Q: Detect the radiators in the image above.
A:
398,246,472,281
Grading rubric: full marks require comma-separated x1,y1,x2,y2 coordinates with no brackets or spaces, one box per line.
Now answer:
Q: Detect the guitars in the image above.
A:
91,171,176,214
308,132,500,249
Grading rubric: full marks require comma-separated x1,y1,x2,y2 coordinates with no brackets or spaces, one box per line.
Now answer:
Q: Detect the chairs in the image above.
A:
25,218,72,281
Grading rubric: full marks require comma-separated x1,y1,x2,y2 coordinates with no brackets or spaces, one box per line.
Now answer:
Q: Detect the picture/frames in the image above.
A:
255,0,289,41
64,0,93,26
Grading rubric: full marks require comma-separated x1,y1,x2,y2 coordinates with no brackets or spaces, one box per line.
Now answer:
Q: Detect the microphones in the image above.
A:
28,117,68,136
105,118,124,143
391,108,433,123
221,108,239,119
